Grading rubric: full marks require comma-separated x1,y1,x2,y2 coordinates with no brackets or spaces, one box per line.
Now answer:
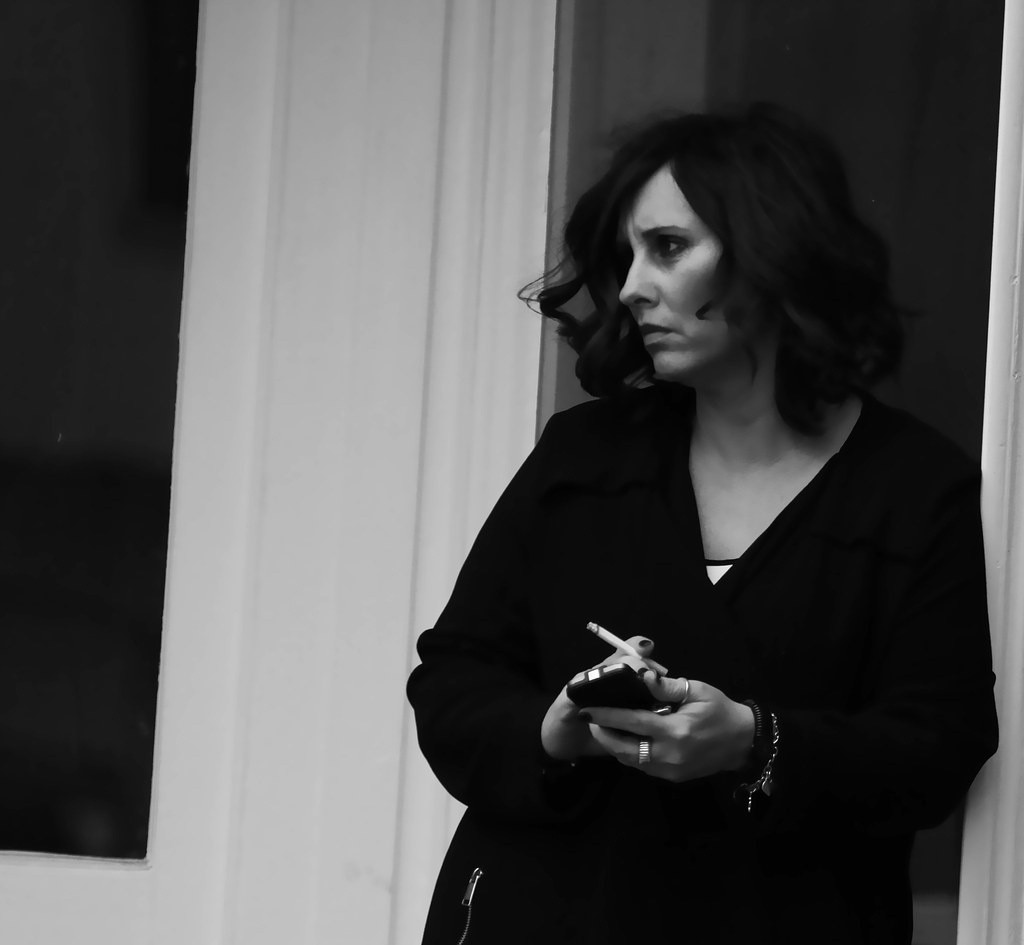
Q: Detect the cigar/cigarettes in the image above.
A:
586,622,669,676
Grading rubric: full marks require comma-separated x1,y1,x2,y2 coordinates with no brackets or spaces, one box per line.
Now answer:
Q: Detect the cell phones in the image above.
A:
565,663,677,739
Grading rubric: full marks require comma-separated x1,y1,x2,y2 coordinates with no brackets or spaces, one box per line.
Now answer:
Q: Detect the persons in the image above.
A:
404,99,1001,944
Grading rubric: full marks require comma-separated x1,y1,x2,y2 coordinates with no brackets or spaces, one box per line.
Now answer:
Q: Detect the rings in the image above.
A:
678,677,691,706
638,735,652,766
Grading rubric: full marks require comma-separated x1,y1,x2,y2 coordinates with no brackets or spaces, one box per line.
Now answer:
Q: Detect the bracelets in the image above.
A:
726,702,780,813
